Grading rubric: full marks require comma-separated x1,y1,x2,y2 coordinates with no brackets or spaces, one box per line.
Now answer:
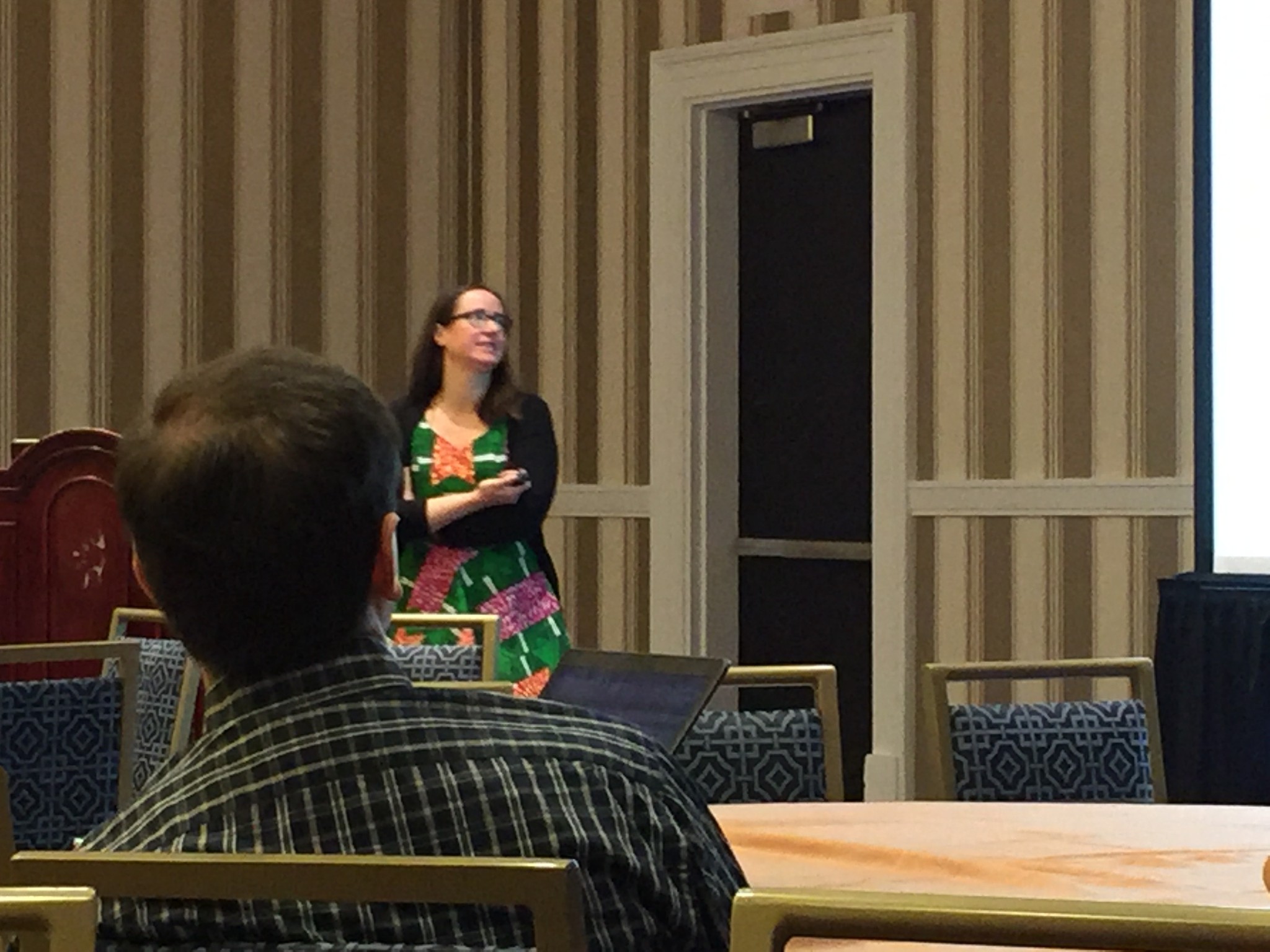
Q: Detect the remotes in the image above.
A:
511,469,528,486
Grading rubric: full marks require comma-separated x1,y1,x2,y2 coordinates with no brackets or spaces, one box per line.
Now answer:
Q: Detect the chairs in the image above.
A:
0,607,1270,952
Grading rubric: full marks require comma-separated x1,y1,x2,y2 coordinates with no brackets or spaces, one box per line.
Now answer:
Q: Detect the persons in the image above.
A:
70,347,748,952
386,287,571,696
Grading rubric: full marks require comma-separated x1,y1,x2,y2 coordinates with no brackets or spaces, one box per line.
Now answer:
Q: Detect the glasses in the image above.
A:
449,308,511,334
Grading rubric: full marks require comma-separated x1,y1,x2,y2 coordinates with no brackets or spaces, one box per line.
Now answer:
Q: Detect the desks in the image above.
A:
707,801,1270,952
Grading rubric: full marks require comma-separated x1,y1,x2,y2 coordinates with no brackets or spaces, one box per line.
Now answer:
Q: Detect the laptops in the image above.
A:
538,648,731,753
